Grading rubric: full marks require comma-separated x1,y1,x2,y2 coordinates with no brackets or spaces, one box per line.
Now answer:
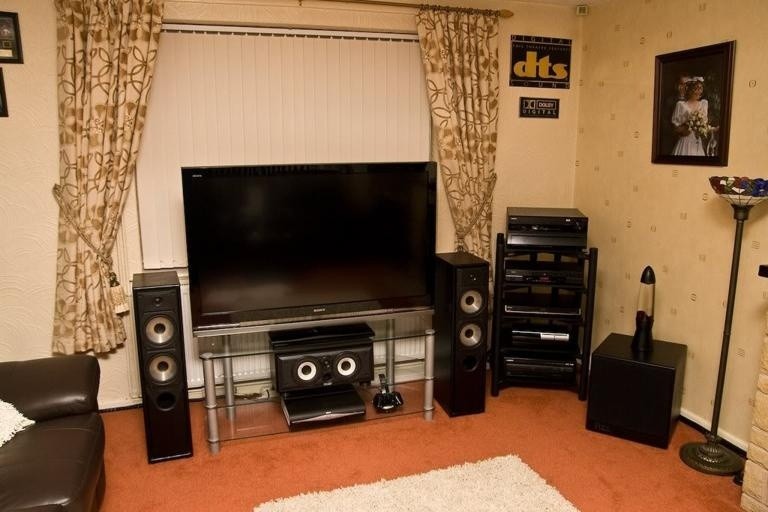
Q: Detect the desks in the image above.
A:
197,316,439,457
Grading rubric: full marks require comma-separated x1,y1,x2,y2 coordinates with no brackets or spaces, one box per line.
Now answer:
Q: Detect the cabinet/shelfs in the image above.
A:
488,232,600,403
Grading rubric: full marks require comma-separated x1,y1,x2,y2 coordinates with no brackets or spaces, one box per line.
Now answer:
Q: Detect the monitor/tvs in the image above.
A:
181,161,437,328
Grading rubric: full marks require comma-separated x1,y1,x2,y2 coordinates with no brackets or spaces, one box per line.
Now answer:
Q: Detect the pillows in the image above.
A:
0,398,36,446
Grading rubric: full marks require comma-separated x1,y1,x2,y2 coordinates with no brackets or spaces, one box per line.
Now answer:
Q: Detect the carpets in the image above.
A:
250,452,580,511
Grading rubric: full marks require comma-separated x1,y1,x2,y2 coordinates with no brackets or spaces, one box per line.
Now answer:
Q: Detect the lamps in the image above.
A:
679,175,768,477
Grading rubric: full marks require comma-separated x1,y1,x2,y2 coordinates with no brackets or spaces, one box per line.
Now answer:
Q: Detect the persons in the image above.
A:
670,75,711,157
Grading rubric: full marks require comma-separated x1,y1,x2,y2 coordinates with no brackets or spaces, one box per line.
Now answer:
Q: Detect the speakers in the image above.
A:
132,270,194,465
434,250,489,418
269,321,376,395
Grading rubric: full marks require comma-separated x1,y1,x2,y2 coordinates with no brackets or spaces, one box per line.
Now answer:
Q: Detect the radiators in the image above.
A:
178,276,435,389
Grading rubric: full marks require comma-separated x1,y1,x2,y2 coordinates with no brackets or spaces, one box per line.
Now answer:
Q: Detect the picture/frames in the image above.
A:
651,39,736,169
0,9,25,65
0,66,9,118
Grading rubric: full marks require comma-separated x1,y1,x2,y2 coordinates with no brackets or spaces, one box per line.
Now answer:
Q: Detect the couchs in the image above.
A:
0,354,107,512
